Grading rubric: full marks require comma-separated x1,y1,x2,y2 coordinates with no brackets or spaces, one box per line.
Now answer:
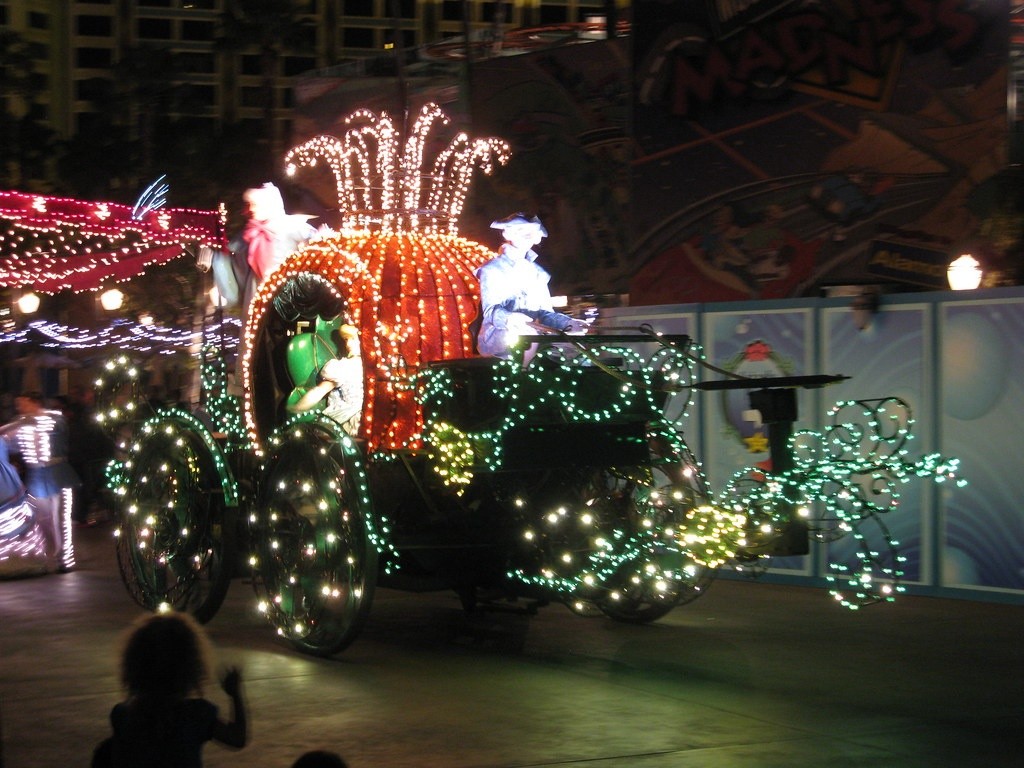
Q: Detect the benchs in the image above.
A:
424,358,667,421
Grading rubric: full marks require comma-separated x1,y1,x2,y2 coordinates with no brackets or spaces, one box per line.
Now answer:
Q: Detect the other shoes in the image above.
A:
75,516,96,529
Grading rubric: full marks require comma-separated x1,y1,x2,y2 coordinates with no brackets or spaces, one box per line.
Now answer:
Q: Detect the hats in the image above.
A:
489,209,549,239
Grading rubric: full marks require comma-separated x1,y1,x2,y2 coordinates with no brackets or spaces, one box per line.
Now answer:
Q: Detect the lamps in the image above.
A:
849,293,879,331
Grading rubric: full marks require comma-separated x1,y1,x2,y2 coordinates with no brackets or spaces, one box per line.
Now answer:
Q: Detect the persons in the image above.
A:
473,213,590,368
1,381,136,572
293,323,364,435
109,611,247,768
